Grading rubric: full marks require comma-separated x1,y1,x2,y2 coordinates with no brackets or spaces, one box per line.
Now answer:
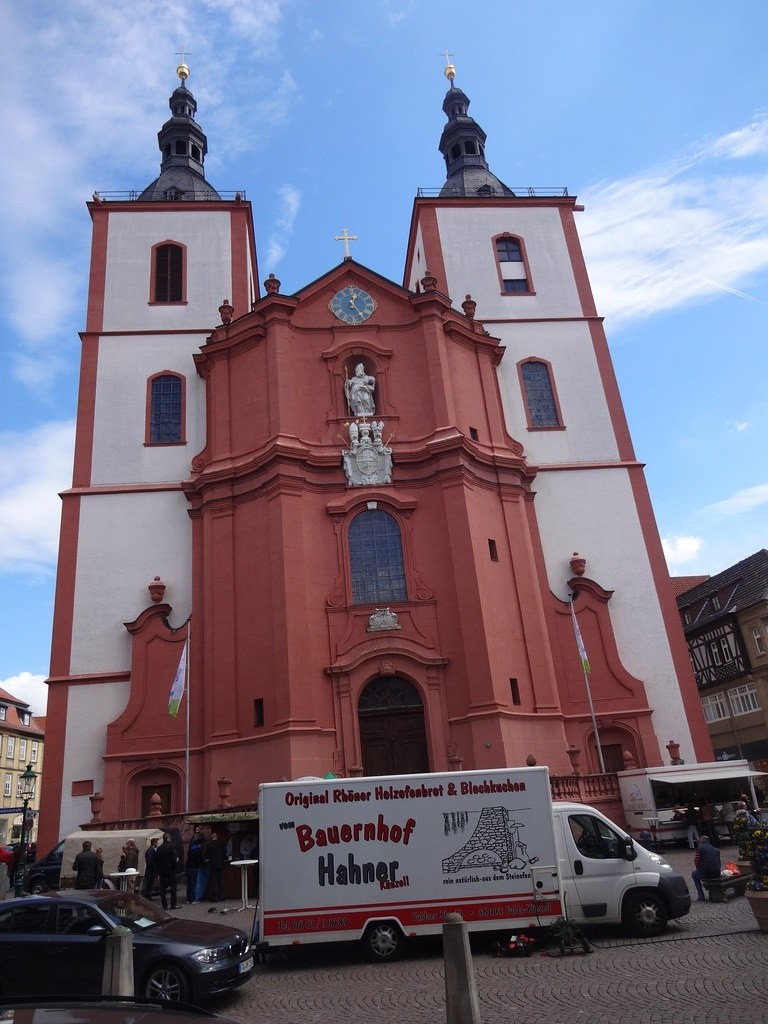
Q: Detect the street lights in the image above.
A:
10,761,39,898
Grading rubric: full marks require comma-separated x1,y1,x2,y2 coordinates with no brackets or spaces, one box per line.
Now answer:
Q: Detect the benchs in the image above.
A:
701,871,752,903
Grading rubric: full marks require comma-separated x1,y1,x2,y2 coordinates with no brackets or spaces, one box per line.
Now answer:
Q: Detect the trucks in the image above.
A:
255,767,696,964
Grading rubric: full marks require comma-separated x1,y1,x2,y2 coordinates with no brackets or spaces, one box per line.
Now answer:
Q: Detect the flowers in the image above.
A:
747,822,768,891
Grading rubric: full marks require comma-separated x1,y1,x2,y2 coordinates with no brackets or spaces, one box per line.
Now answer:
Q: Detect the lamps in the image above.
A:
440,48,456,80
173,45,193,80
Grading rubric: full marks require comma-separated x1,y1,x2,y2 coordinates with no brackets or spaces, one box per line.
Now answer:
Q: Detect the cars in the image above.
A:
24,838,66,894
1,891,255,1011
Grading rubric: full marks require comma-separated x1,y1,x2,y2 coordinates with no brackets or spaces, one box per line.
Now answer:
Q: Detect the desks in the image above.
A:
108,872,140,917
230,859,259,912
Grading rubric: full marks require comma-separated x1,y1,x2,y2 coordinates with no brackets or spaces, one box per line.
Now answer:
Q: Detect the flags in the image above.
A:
569,600,590,673
168,641,187,718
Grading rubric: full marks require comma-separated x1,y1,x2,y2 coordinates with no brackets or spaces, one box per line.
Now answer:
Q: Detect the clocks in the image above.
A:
326,284,378,325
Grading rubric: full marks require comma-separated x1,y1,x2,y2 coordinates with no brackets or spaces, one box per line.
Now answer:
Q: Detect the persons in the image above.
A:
345,362,375,418
6,841,36,890
673,794,758,848
73,827,258,909
349,421,384,449
691,835,722,902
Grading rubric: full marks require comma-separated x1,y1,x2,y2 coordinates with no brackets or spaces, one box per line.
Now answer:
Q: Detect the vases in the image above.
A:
744,890,768,933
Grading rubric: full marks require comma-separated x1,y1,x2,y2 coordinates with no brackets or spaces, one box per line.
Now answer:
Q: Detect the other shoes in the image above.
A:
697,897,705,900
191,899,200,903
171,904,181,910
163,906,168,909
